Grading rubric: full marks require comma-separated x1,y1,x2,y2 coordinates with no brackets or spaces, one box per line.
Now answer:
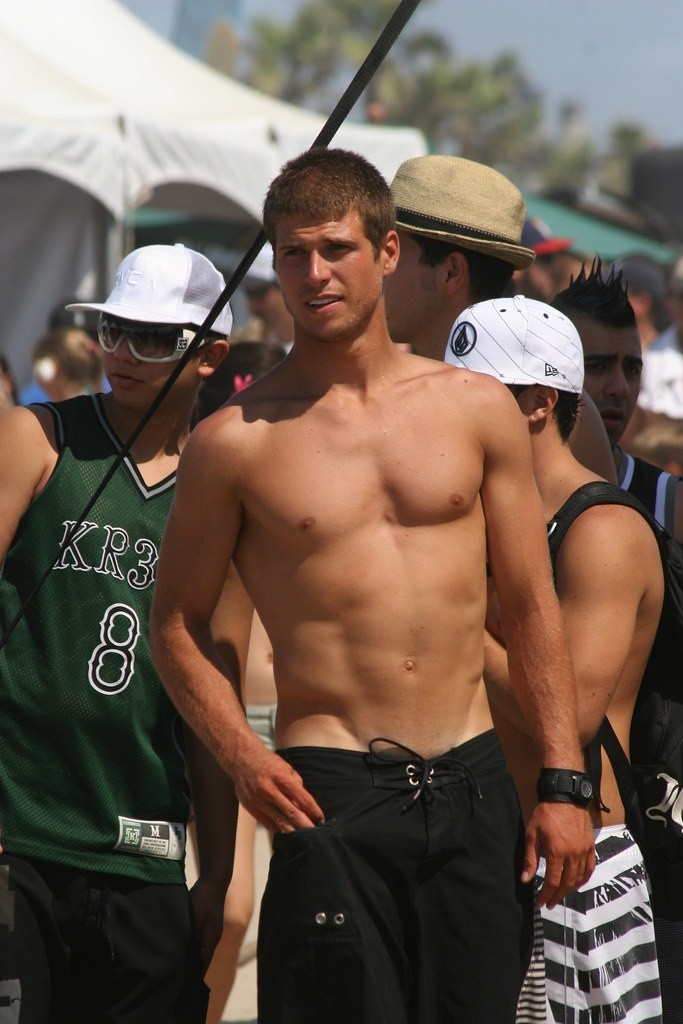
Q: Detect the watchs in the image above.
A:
538,769,596,806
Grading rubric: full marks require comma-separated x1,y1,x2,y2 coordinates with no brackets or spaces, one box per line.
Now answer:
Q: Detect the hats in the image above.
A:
520,219,571,255
65,243,233,336
444,295,584,400
390,156,536,270
52,299,99,333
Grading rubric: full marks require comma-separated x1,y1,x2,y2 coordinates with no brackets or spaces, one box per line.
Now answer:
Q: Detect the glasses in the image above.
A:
506,384,530,399
97,312,228,363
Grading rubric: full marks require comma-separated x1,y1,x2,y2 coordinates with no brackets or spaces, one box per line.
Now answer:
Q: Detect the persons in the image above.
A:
150,147,595,1023
2,151,682,1024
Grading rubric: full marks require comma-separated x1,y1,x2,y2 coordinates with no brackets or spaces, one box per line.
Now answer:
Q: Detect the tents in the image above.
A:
1,0,432,400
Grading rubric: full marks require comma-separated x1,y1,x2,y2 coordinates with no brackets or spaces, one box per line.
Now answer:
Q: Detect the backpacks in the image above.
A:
545,481,683,921
